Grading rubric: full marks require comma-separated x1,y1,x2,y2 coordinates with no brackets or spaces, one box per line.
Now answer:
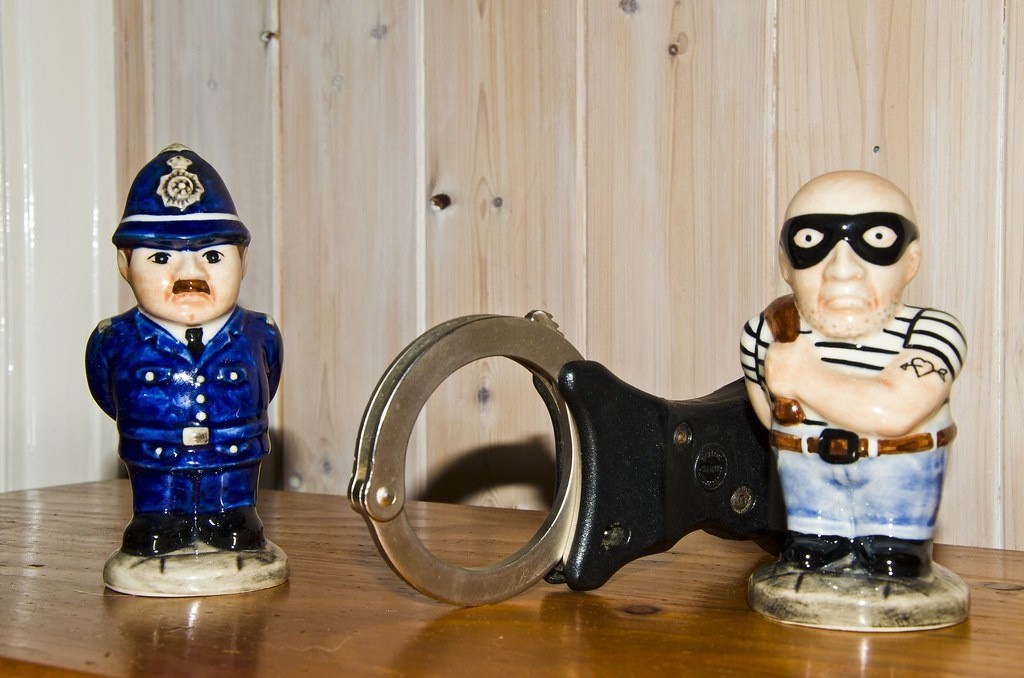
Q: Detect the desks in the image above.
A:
0,478,1024,678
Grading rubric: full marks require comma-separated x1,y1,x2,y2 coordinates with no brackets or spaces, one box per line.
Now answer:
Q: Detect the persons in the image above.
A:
740,170,968,576
84,146,284,554
